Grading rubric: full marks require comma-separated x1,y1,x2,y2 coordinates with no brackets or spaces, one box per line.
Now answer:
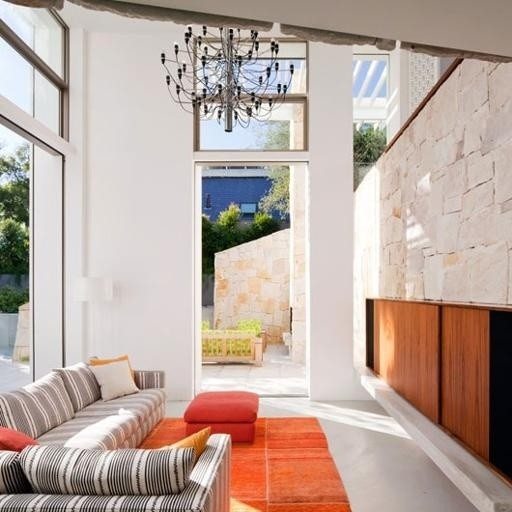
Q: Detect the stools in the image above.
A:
184,391,260,443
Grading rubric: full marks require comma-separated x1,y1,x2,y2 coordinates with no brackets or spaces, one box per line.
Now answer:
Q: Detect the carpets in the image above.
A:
135,417,352,512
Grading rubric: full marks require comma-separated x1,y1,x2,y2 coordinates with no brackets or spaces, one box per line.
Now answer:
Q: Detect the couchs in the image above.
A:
0,355,232,512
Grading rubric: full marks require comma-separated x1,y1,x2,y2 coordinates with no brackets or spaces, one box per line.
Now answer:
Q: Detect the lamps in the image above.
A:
159,21,295,132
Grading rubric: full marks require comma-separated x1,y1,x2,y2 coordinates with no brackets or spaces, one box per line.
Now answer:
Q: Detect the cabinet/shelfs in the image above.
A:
362,297,511,489
